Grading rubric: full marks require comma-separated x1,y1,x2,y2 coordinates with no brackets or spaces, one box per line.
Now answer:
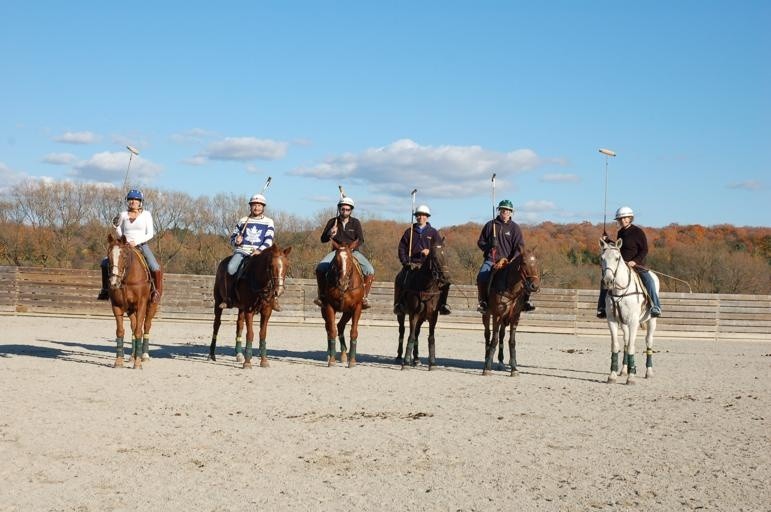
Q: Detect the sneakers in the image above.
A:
522,300,536,311
314,297,322,307
218,296,233,309
393,302,400,313
596,308,606,318
271,297,283,312
362,297,371,309
439,304,451,315
650,306,662,317
477,300,488,314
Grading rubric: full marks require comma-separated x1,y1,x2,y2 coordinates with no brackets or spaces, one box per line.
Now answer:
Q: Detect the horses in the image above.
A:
598,238,662,387
315,238,372,369
106,233,164,369
476,245,542,378
394,235,453,372
207,242,292,368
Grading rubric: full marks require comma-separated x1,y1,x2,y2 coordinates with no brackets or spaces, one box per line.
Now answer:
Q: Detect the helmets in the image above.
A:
126,189,145,201
496,199,514,212
612,206,634,220
248,194,267,206
413,205,431,217
337,198,355,209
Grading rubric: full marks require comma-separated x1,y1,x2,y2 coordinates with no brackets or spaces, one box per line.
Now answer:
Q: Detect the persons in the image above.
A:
393,205,453,316
476,199,537,315
595,206,663,319
218,194,286,313
313,197,376,309
97,189,164,304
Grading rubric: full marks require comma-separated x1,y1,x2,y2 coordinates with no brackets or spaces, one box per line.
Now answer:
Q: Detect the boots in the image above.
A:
150,270,162,304
97,264,110,301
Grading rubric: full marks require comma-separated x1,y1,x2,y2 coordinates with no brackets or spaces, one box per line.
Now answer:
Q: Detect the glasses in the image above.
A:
341,208,350,211
